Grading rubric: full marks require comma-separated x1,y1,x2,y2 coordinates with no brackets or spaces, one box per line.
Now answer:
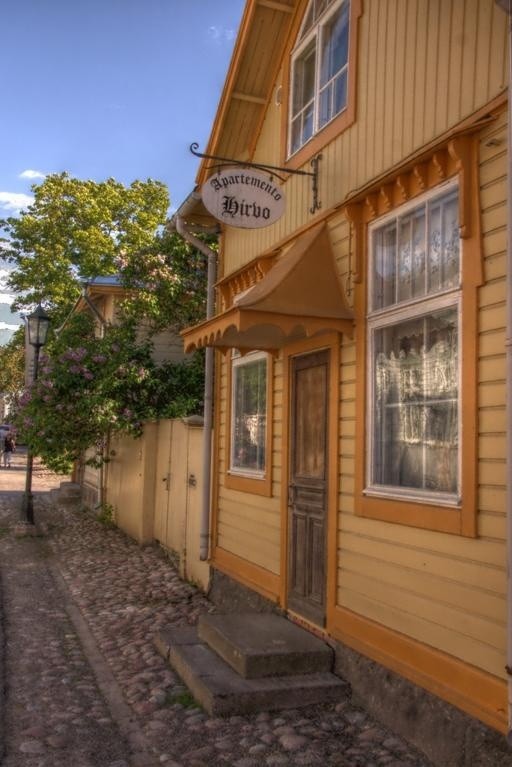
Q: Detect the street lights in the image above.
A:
21,296,52,524
